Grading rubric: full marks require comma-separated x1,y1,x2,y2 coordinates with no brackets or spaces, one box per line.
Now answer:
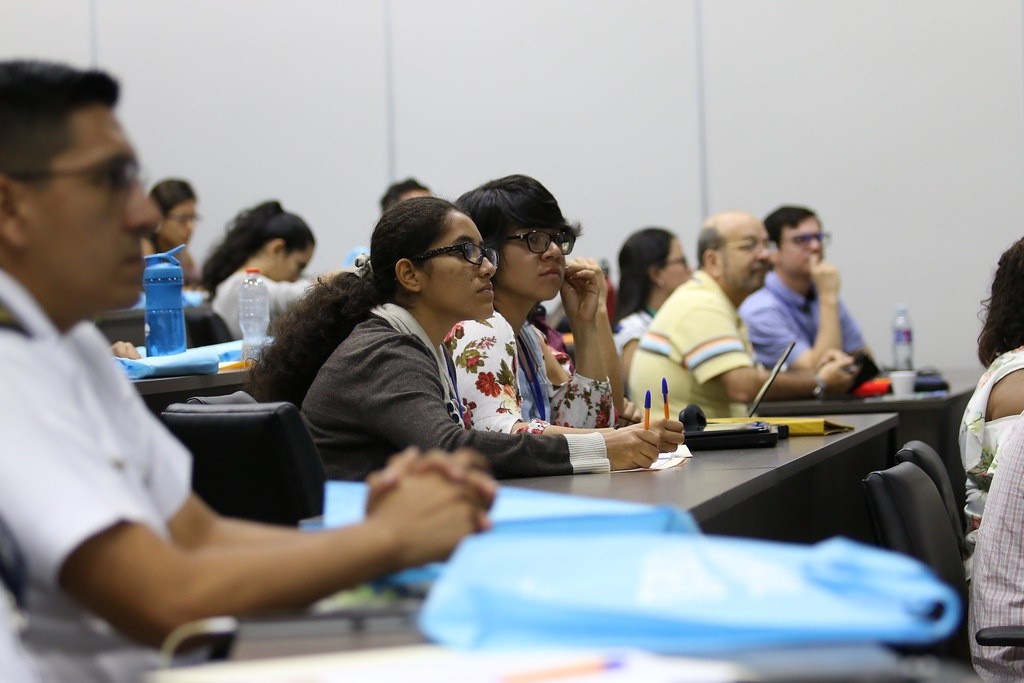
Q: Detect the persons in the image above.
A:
0,60,496,683
738,207,868,373
247,197,659,484
957,237,1024,683
628,212,854,421
202,201,316,340
445,175,685,453
612,230,692,383
383,179,429,212
141,179,198,290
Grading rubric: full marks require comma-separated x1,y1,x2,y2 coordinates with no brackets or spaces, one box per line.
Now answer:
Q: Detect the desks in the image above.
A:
131,365,250,414
493,412,902,547
95,309,145,347
747,375,975,539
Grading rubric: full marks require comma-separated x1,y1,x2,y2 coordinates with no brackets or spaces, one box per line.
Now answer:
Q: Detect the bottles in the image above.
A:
893,308,914,372
238,268,270,361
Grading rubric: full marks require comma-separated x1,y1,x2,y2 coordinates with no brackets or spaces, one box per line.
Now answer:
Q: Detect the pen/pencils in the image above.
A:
643,389,652,431
661,378,674,424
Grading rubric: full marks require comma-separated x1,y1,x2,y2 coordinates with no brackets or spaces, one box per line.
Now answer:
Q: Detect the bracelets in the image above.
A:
817,377,824,398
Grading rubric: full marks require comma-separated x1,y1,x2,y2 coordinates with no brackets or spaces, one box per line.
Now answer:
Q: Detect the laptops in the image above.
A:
747,340,796,416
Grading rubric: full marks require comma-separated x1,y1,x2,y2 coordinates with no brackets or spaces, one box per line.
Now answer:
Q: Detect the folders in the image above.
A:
706,416,854,435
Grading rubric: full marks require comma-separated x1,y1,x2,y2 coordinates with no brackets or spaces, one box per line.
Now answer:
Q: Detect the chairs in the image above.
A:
189,391,259,407
181,308,234,350
161,401,329,528
862,461,1024,674
893,438,966,563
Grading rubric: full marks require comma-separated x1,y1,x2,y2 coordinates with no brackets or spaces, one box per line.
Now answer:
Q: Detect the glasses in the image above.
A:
8,155,139,193
490,230,576,256
393,240,501,270
786,233,830,246
167,212,199,226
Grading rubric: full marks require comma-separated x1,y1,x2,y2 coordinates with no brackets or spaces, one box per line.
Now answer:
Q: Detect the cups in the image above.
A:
890,372,916,395
143,244,187,357
